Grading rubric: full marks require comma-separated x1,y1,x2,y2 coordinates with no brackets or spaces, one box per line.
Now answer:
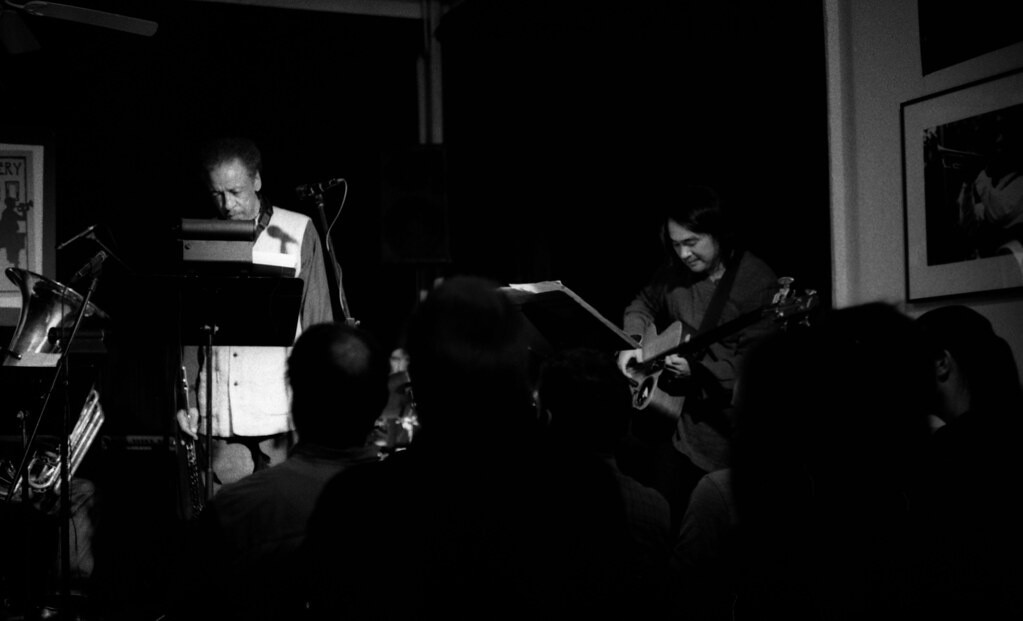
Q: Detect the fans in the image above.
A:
0,0,158,55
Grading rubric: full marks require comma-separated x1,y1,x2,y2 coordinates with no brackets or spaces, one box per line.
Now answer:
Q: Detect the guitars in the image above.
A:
630,275,819,435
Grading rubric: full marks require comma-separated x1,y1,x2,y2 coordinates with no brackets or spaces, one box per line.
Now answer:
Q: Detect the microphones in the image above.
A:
57,224,97,249
70,250,107,283
296,177,344,198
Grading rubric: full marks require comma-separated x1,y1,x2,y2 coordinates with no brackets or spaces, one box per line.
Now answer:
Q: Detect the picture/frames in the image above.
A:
0,140,56,325
899,67,1023,305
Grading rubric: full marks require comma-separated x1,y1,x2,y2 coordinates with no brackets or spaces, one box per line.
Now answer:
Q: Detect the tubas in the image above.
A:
0,265,106,502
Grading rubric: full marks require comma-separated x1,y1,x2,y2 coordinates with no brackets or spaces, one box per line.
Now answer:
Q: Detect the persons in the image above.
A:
178,276,1023,621
958,117,1023,256
612,190,780,511
183,148,335,494
